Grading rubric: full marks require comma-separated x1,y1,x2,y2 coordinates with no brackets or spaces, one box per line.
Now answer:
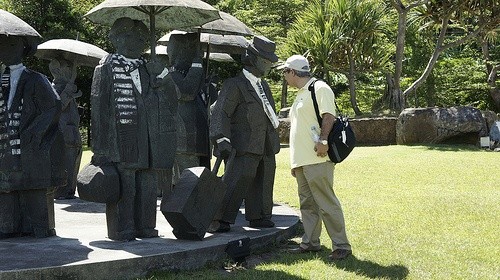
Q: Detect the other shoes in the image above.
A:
289,245,320,254
329,248,351,259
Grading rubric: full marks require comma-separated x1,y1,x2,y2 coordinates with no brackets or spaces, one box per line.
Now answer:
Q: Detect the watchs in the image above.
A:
319,140,329,145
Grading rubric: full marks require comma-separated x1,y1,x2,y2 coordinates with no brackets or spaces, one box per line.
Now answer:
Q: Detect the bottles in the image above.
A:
311,126,327,158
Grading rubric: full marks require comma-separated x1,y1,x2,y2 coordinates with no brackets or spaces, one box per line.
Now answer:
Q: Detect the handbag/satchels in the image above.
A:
319,117,356,164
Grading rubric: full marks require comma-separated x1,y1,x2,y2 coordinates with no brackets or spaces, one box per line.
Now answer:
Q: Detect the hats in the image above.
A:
277,55,310,72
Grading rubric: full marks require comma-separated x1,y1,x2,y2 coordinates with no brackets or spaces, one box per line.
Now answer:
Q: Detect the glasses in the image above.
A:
283,70,289,76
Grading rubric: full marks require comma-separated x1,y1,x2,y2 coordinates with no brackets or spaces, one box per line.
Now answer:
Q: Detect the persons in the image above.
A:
284,55,351,259
0,34,60,237
158,34,217,194
208,36,282,231
90,15,162,240
47,60,82,201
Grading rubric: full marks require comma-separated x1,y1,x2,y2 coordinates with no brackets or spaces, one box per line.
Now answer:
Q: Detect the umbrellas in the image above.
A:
0,9,44,54
35,32,109,66
84,1,223,61
158,29,251,56
195,11,254,37
147,45,235,63
